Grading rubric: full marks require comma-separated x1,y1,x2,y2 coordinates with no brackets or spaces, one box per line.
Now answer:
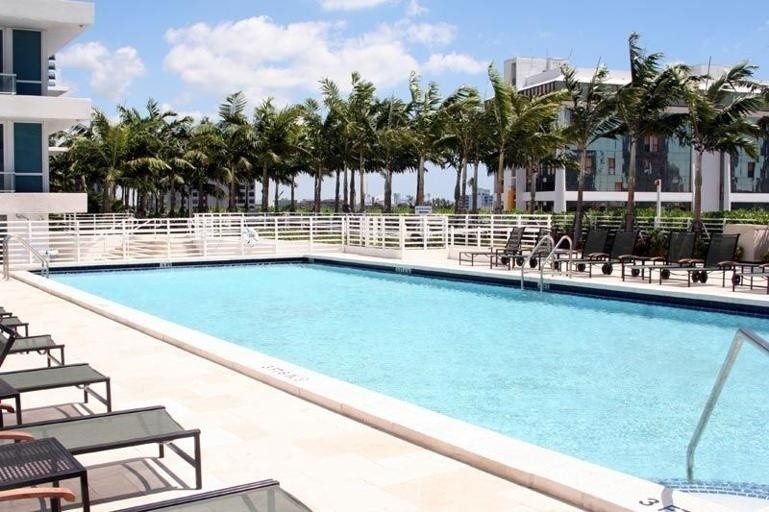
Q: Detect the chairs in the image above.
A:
0,317,29,338
1,361,114,428
0,323,65,368
4,404,202,510
457,225,768,290
0,434,309,510
